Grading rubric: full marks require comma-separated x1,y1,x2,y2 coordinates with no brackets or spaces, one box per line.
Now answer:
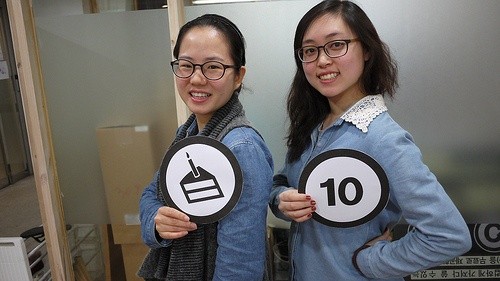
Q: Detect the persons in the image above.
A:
138,13,274,281
269,3,474,281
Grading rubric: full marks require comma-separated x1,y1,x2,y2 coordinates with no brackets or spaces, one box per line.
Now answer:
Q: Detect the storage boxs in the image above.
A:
97,116,161,246
121,245,149,281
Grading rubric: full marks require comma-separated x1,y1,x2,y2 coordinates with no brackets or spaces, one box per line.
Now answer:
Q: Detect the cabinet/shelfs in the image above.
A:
0,222,105,280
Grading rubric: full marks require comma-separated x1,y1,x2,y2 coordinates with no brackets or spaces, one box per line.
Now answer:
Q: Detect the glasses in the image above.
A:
170,59,236,81
298,39,363,63
